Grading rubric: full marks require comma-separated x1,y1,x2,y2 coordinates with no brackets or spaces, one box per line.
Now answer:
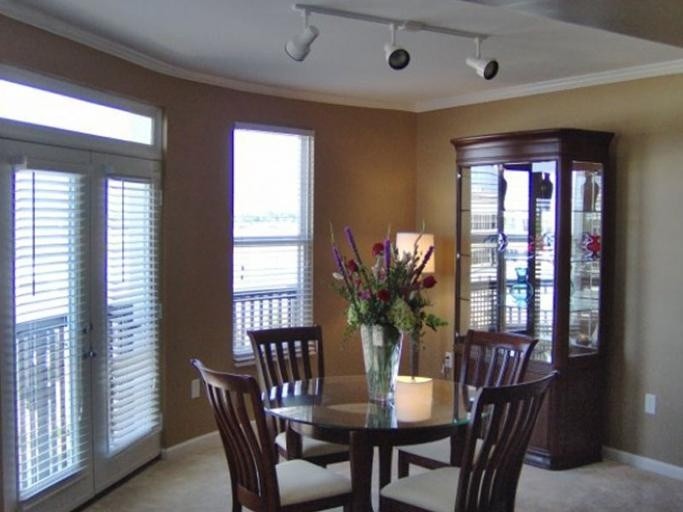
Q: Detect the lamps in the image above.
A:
393,232,434,375
283,4,499,83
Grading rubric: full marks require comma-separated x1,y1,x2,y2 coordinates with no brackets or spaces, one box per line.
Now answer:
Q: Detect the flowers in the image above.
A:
318,217,450,353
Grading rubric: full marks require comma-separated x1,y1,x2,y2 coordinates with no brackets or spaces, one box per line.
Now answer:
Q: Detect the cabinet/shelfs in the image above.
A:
449,127,615,471
